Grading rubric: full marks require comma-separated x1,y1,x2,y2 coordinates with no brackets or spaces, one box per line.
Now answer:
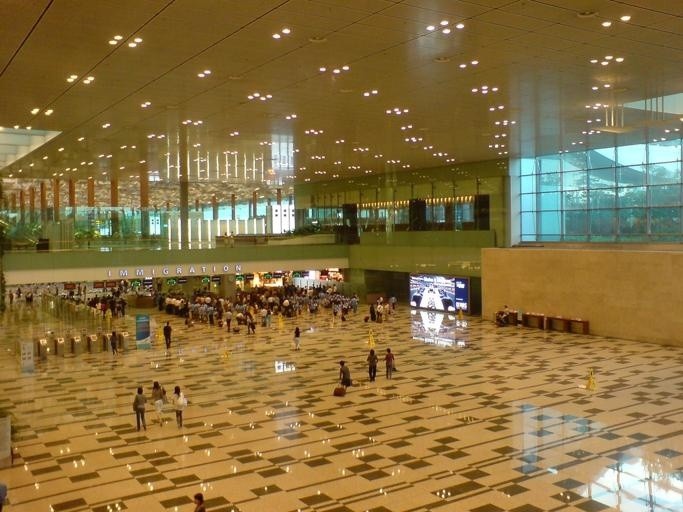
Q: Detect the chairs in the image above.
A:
37,238,49,251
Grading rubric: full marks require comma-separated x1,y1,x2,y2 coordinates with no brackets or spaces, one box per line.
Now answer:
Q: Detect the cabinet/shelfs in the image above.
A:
496,311,589,335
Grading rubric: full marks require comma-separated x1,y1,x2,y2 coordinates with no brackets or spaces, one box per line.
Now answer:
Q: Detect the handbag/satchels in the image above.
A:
392,368,396,371
163,394,168,404
184,398,188,406
233,326,240,331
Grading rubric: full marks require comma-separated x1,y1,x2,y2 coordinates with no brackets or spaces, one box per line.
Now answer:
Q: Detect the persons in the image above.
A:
503,304,510,324
370,295,397,323
110,330,119,357
150,287,320,334
171,386,184,428
8,283,87,312
163,321,172,350
293,325,301,352
229,232,236,248
322,284,359,321
383,347,395,380
152,381,166,428
458,307,464,327
338,359,351,392
223,231,229,248
365,349,379,383
85,280,128,318
133,387,147,432
192,493,205,512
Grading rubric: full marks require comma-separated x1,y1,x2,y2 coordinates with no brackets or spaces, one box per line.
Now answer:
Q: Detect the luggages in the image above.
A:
218,319,223,327
334,381,346,396
365,313,371,322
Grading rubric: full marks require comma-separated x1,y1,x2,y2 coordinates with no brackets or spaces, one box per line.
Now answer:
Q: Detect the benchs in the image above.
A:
10,239,29,249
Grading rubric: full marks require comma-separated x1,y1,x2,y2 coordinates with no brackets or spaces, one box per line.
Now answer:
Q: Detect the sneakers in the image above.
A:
370,377,375,381
